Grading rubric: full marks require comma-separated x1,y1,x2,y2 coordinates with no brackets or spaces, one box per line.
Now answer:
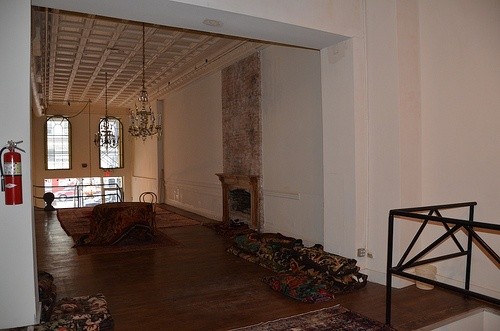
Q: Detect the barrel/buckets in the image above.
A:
415,264,436,290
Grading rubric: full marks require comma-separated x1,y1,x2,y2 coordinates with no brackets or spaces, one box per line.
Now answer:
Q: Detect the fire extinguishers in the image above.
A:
1,140,26,205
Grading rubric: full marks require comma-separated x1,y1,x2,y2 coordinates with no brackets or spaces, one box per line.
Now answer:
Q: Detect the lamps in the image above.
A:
94,72,120,149
125,22,163,139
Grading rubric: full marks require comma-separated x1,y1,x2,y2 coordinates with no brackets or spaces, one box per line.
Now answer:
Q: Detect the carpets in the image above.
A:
224,301,399,331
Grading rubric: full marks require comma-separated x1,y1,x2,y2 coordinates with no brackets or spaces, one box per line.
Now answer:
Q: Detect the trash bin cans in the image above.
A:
415,264,437,291
